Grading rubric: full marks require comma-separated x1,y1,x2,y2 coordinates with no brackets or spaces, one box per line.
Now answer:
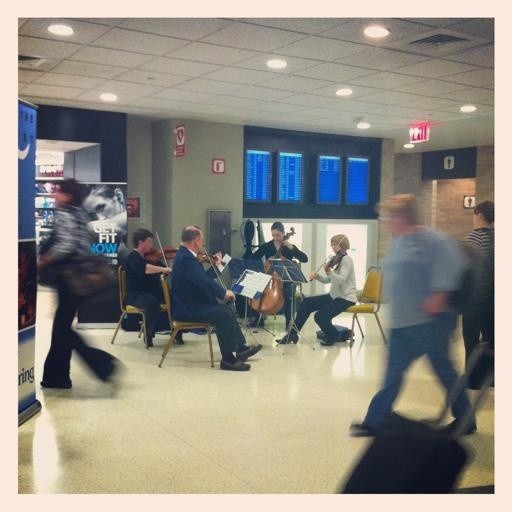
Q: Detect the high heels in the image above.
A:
321,333,340,345
276,334,298,344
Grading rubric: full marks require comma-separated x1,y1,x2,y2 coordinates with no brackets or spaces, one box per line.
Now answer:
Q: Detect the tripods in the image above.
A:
276,282,315,356
236,297,275,344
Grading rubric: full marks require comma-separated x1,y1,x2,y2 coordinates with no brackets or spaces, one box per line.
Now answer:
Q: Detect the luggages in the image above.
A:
341,342,493,491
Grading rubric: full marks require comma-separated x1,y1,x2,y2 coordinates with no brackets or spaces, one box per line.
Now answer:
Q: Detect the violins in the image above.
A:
145,246,179,265
197,250,226,267
325,249,347,270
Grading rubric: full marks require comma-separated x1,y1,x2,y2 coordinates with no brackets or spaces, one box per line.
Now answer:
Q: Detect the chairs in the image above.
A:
341,266,387,350
111,263,215,370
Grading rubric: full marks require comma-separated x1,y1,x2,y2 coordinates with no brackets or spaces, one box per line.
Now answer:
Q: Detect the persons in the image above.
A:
348,193,478,440
123,229,186,349
275,234,356,346
245,222,308,333
458,200,493,389
167,223,262,371
36,179,130,396
74,184,128,244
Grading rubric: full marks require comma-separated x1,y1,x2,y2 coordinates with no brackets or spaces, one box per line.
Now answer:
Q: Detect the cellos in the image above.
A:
248,225,296,315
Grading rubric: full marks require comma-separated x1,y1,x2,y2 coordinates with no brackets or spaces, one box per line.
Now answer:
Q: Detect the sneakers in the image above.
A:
447,419,476,435
221,357,250,371
236,344,262,361
247,319,264,327
143,325,153,347
174,332,184,344
350,424,375,437
40,378,71,389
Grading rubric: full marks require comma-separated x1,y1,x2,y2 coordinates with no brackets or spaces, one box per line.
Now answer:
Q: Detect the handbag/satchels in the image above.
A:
447,239,484,317
61,251,116,297
38,236,77,288
317,325,354,342
